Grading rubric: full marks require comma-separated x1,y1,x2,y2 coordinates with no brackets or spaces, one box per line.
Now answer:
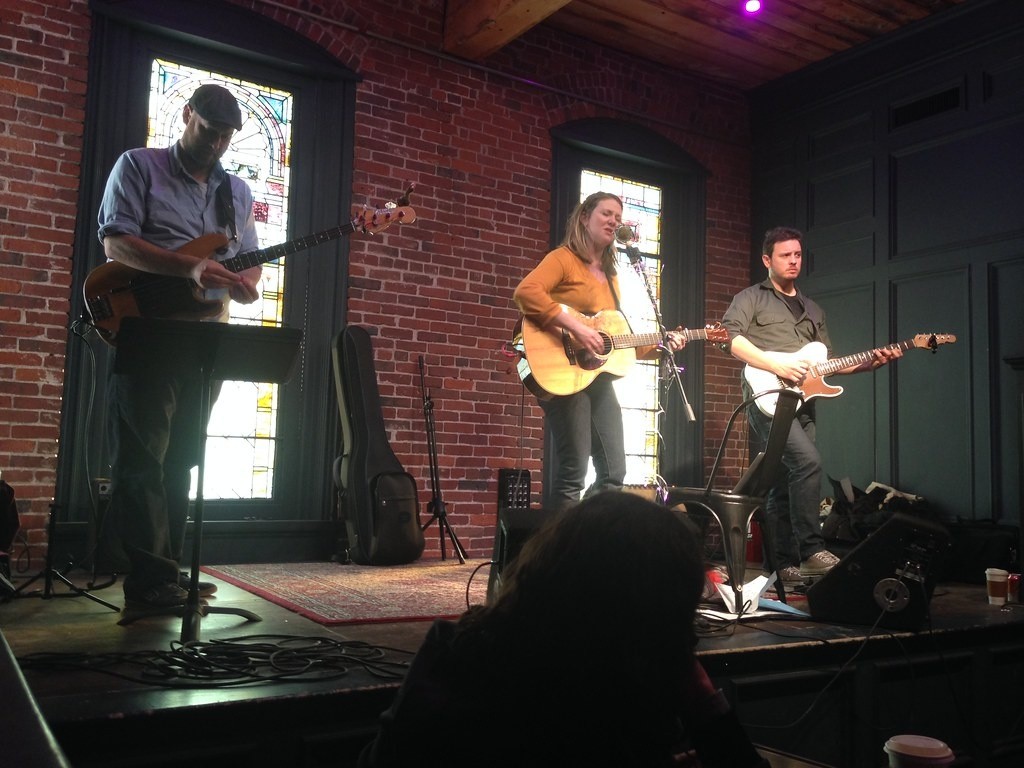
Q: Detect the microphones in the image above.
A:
616,226,643,268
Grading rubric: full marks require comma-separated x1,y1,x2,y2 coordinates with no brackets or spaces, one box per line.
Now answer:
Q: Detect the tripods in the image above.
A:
418,355,469,563
3,504,122,612
111,317,304,648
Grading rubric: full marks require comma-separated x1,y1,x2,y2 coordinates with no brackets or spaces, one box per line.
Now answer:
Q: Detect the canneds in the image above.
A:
1007,574,1022,602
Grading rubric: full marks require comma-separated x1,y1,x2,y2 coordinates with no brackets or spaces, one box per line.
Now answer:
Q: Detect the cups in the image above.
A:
985,568,1009,607
883,735,955,768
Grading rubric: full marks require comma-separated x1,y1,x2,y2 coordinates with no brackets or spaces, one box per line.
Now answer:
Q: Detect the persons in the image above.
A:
353,489,776,768
95,85,262,618
719,223,903,585
514,192,690,510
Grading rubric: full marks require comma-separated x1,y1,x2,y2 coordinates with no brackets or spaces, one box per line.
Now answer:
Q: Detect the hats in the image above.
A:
188,84,242,131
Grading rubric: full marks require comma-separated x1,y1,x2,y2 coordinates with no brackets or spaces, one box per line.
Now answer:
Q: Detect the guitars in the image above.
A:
743,329,958,422
512,301,733,403
84,201,417,350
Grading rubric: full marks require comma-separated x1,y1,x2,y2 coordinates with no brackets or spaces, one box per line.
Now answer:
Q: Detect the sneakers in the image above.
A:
800,550,840,577
761,565,805,586
123,569,218,617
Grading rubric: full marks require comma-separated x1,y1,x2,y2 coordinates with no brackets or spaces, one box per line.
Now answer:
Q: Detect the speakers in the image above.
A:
805,510,952,634
485,508,554,607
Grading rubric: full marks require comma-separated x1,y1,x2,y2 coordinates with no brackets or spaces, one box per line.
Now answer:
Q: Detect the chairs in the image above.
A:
655,387,806,616
